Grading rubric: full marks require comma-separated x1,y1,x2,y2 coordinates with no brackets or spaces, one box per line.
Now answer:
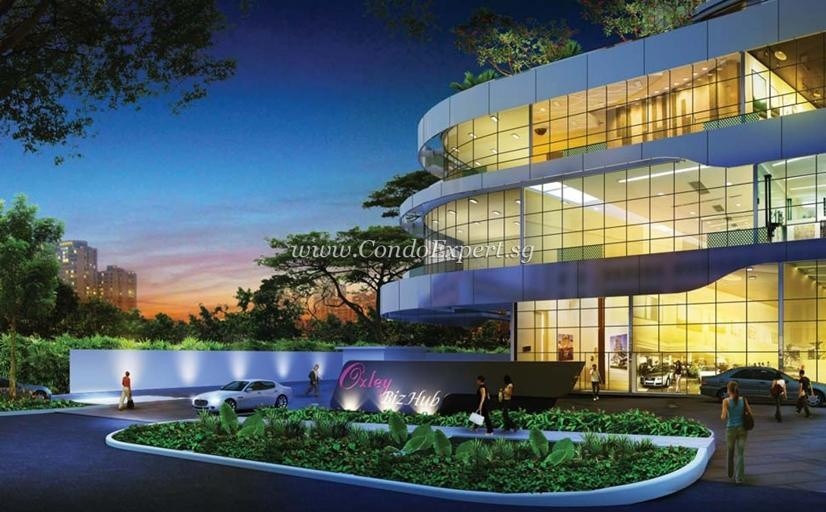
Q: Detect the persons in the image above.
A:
720,379,753,484
558,340,564,360
469,375,494,435
671,359,683,393
770,371,788,424
587,363,602,403
567,339,573,360
793,368,815,420
117,371,131,411
304,363,320,397
498,375,516,434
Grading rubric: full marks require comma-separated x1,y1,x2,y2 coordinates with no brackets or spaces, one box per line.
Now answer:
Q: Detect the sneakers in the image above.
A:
736,479,744,484
728,471,736,481
796,410,812,418
776,415,783,422
502,424,518,434
593,396,599,401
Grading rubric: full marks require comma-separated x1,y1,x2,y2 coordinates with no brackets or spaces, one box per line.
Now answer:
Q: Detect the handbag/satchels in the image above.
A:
469,409,485,426
770,384,784,398
743,398,754,431
127,400,134,408
500,398,514,411
